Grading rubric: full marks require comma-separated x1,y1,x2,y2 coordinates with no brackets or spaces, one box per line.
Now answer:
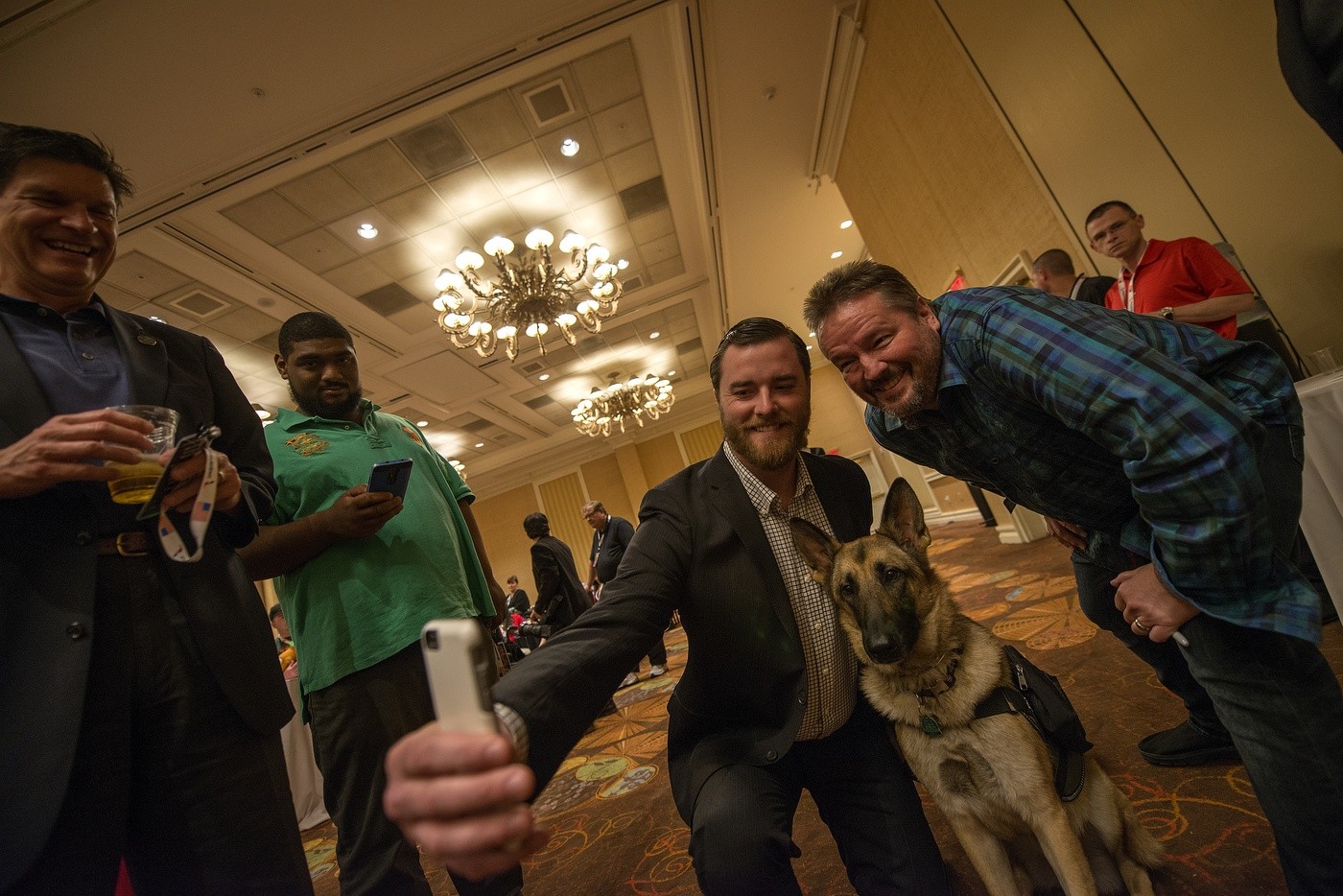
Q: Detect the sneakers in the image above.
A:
1136,717,1242,768
594,699,618,719
649,663,668,679
582,722,595,736
617,672,639,691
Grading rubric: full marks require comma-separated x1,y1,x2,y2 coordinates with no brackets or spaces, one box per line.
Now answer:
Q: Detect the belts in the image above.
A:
97,529,155,558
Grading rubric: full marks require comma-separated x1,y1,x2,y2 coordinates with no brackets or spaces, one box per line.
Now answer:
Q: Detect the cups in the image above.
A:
99,405,180,504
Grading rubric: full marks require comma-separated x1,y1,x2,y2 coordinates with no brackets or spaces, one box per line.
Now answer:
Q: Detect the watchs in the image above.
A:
1162,305,1174,320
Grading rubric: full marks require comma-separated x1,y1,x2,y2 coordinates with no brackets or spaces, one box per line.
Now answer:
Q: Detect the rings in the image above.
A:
1134,619,1150,632
218,470,226,482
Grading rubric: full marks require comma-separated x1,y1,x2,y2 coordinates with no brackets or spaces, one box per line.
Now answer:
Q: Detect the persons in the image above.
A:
0,120,316,896
268,601,297,653
1030,250,1114,308
582,501,669,689
505,573,530,615
1086,200,1256,342
236,310,518,896
522,510,593,635
802,259,1342,896
376,318,957,896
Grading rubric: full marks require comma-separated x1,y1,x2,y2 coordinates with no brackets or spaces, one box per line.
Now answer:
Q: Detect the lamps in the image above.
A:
567,372,676,434
431,224,627,364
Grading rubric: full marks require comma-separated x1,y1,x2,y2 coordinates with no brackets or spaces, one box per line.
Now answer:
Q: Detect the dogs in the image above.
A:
787,476,1166,896
